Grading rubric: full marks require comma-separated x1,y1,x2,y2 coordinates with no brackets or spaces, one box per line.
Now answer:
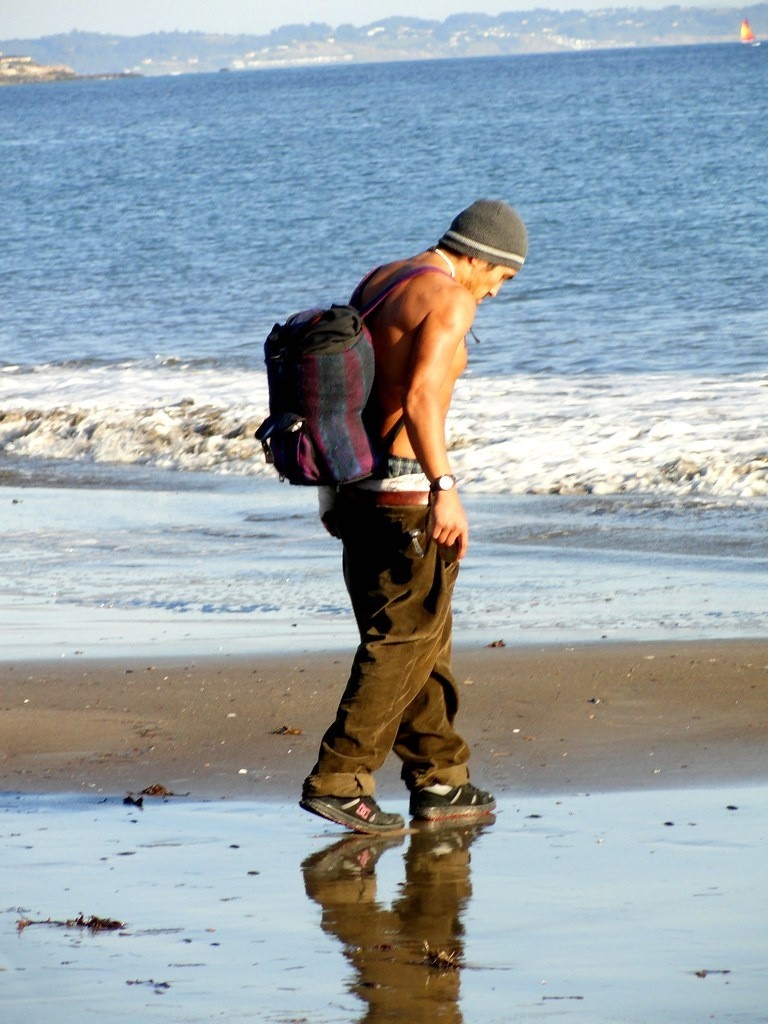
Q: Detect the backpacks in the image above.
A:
254,266,456,486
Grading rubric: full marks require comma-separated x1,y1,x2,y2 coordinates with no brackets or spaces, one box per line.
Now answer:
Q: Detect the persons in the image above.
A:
299,200,529,833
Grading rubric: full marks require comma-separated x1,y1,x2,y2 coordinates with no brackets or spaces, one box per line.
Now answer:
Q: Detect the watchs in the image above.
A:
430,474,456,492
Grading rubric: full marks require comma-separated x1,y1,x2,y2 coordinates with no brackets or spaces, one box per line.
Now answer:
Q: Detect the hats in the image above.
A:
438,198,529,272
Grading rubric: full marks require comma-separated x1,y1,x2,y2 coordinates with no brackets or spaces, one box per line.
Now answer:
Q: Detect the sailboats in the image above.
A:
740,18,763,47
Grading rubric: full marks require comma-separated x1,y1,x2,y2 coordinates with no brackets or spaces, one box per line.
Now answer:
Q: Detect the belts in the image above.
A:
329,484,429,508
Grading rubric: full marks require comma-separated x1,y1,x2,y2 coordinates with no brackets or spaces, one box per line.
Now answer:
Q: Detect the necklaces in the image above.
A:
426,246,456,277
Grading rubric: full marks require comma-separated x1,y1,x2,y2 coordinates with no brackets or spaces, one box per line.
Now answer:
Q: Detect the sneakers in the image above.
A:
299,795,404,836
410,783,496,819
